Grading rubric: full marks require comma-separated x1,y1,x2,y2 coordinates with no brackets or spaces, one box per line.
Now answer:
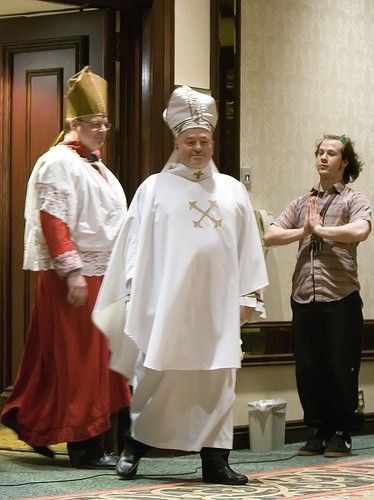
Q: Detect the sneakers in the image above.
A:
298,428,331,456
324,430,352,457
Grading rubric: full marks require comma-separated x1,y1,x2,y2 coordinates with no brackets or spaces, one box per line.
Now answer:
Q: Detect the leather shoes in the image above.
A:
68,444,120,470
116,444,140,479
202,464,249,485
33,446,54,457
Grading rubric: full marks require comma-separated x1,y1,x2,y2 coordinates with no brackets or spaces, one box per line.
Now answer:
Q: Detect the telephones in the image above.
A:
254,210,274,246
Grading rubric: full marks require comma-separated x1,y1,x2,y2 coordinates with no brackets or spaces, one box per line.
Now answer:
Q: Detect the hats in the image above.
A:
65,66,109,119
162,86,218,137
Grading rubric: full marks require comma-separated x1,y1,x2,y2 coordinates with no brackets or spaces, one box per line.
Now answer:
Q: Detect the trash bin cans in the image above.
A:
248,399,287,452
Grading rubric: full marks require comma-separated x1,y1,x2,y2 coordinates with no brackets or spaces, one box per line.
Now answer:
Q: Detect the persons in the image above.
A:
263,134,374,458
0,65,128,470
116,83,269,485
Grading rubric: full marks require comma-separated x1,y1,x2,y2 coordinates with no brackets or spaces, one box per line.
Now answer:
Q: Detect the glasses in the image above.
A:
77,119,112,130
